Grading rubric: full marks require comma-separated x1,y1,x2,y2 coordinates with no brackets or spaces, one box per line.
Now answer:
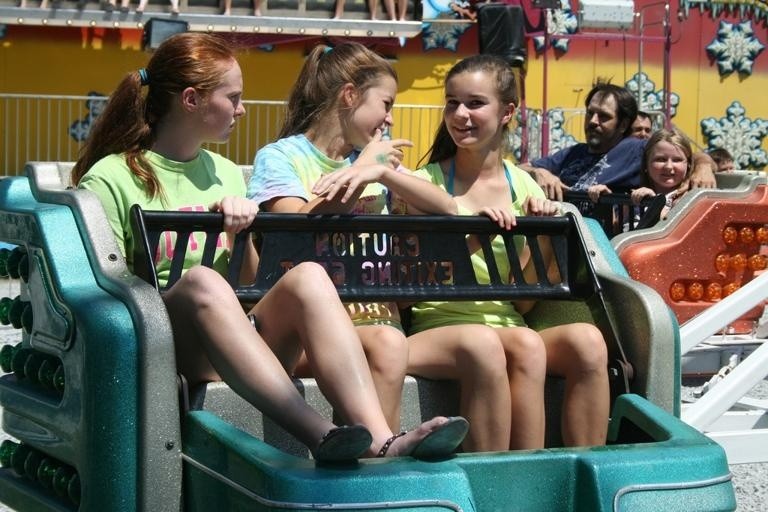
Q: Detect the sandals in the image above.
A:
316,425,373,460
376,417,470,458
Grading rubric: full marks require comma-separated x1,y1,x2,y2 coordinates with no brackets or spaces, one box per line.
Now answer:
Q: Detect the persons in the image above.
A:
71,31,470,464
388,54,611,449
246,42,512,452
515,76,735,241
